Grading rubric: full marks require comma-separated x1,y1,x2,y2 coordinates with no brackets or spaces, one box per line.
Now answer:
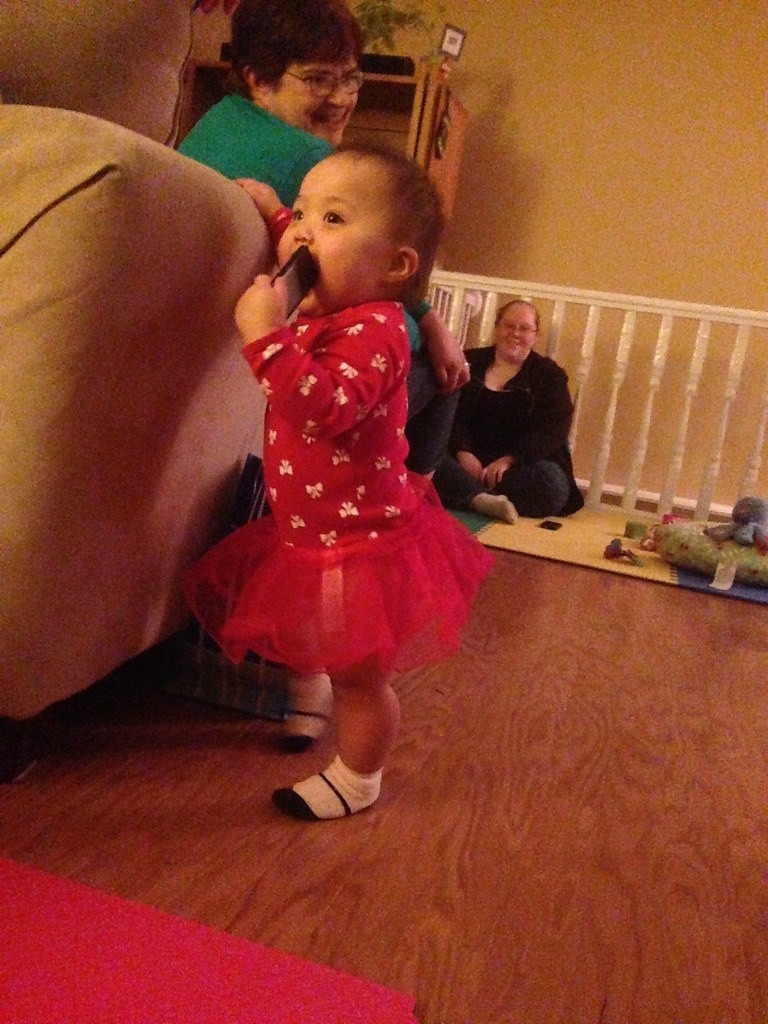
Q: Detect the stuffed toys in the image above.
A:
699,495,768,547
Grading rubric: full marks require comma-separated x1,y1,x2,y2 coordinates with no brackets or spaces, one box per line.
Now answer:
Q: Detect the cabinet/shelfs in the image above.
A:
175,55,472,250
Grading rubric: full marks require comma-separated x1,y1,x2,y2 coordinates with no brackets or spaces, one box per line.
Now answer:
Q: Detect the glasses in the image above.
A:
499,321,539,333
283,66,366,97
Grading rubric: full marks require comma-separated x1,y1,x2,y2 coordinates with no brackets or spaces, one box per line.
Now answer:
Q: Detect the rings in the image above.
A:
464,362,469,367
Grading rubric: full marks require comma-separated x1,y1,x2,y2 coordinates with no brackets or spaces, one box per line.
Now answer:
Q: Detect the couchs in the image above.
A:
0,0,271,778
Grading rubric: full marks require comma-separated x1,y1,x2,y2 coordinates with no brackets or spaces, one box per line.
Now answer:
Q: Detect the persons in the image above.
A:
180,2,472,487
428,299,588,525
180,139,495,821
